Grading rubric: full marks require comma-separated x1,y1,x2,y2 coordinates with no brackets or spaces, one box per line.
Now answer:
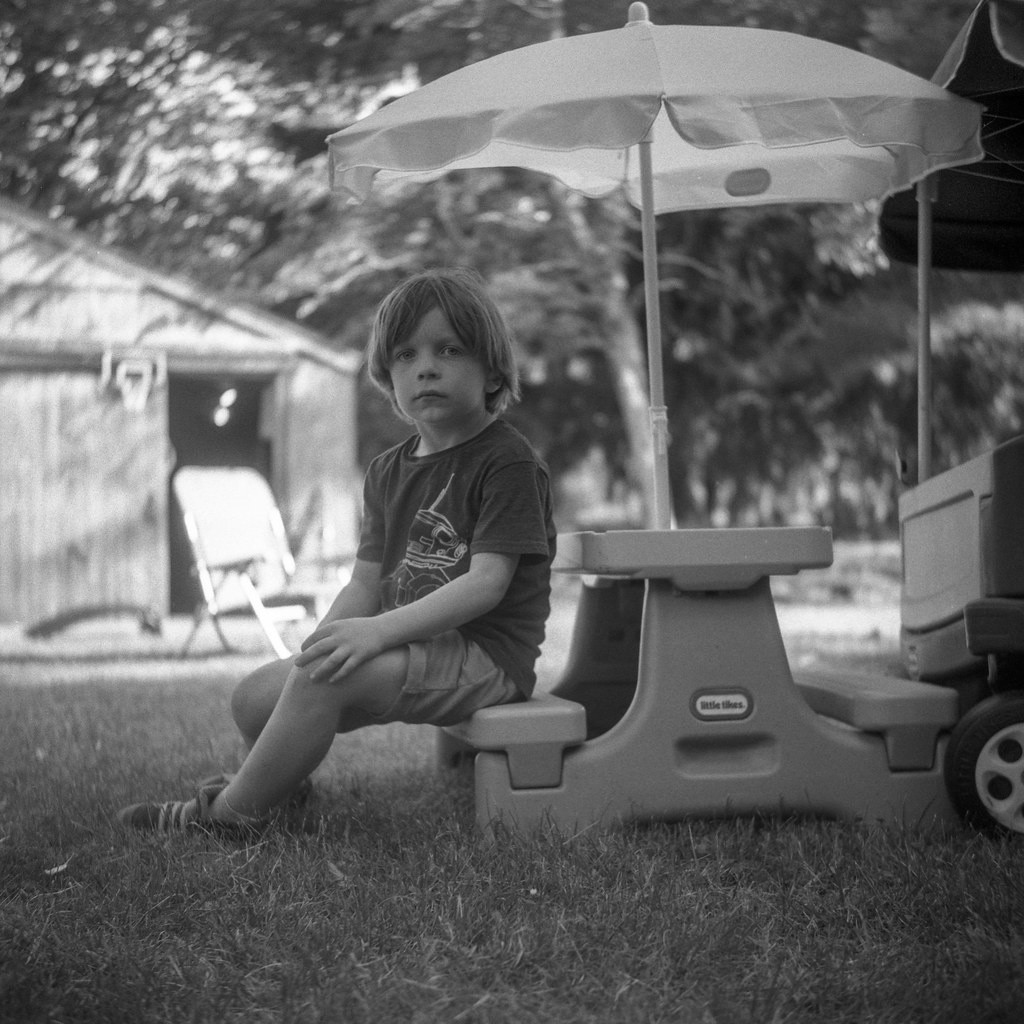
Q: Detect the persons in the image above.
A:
114,269,557,845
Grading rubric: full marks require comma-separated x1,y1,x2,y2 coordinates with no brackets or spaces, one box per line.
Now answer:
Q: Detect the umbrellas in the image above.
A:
332,2,985,524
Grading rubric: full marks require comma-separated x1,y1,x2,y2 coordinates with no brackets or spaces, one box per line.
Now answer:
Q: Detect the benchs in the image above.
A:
434,685,587,789
788,668,961,770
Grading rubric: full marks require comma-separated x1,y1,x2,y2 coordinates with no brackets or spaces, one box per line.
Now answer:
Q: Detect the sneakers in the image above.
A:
118,770,313,844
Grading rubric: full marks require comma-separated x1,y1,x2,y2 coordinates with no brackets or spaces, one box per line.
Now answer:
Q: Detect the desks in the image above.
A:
444,527,960,837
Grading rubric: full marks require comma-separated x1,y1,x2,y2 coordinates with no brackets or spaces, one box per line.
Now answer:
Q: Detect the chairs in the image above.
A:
170,466,338,655
